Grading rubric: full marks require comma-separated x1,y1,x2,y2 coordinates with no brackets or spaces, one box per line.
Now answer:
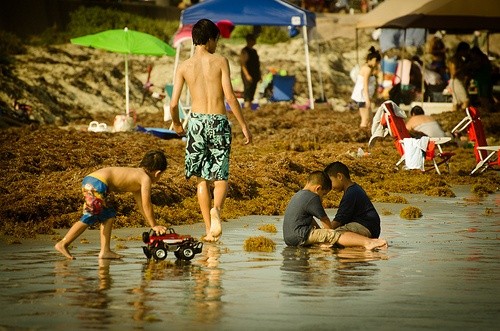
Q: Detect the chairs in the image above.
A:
271,74,296,101
140,64,165,110
383,102,451,175
162,83,194,132
451,106,500,175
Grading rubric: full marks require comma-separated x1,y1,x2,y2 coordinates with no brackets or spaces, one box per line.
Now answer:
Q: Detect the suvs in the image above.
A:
142,228,203,262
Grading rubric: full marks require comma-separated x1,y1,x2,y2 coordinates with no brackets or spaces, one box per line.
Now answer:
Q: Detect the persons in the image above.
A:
54,149,168,260
282,160,388,251
169,18,253,242
240,33,262,110
350,35,500,138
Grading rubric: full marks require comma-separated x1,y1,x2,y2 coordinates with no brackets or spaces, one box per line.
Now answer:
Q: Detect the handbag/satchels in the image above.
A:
350,65,360,83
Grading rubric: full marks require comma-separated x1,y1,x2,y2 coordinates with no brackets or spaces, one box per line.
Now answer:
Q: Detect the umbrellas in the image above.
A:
70,26,177,116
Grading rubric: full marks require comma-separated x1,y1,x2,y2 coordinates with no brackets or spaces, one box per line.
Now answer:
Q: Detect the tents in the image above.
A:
354,0,500,102
172,0,325,109
